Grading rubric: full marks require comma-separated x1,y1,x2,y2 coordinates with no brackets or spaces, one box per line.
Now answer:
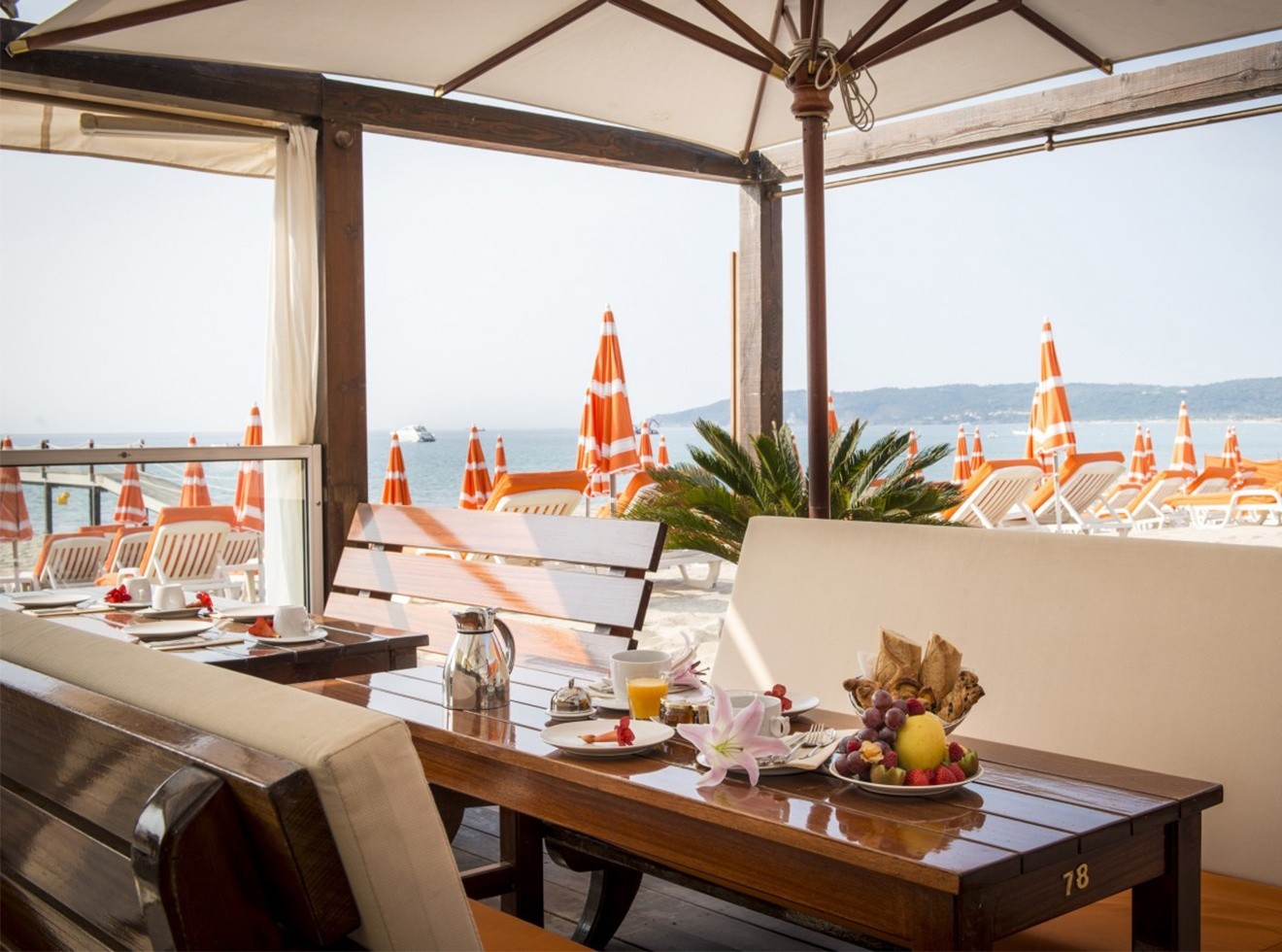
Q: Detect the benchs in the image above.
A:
695,514,1282,952
323,501,661,670
1,611,589,951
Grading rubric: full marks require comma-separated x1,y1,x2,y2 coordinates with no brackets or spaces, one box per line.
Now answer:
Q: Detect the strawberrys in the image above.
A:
880,750,898,770
906,698,925,717
904,741,965,786
846,740,861,754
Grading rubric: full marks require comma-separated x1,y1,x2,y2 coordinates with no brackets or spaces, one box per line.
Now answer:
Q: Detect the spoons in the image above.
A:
800,726,836,759
150,628,228,647
30,600,98,614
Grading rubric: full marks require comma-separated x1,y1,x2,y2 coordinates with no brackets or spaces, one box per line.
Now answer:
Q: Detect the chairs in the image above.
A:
1091,483,1138,529
593,473,723,590
929,457,1044,532
397,468,590,606
1161,481,1281,531
1183,466,1228,496
1105,471,1192,535
1007,451,1133,538
0,508,265,605
1231,465,1265,490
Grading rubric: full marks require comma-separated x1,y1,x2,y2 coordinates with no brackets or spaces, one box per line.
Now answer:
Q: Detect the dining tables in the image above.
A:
309,647,1222,952
40,596,420,687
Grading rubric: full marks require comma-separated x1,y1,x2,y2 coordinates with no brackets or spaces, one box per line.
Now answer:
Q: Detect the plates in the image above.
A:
539,719,675,758
710,689,819,718
696,749,807,776
98,597,151,608
8,593,90,608
545,708,597,721
220,605,274,623
245,630,327,643
588,692,629,710
125,619,215,639
136,607,201,618
829,756,984,796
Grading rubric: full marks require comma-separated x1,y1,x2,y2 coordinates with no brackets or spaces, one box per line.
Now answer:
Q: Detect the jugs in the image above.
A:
442,606,516,710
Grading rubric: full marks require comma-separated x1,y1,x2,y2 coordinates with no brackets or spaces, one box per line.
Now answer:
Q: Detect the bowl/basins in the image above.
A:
611,651,672,703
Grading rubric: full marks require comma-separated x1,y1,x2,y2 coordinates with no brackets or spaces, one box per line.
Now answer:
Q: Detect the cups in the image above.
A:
120,578,151,602
152,585,187,611
272,604,315,636
709,694,791,737
625,672,670,722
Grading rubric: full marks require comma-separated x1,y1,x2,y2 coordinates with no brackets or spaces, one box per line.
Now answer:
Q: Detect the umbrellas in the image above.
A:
492,434,508,486
577,302,640,516
828,394,841,442
1231,424,1244,472
1145,429,1160,477
1222,427,1233,473
1032,314,1079,532
638,421,658,473
657,436,669,471
1127,422,1152,484
382,432,410,507
1028,387,1055,475
0,434,36,574
181,435,212,507
111,464,148,525
908,429,924,482
8,0,1279,519
234,401,266,532
459,427,494,512
1170,398,1199,494
952,423,972,484
970,427,987,472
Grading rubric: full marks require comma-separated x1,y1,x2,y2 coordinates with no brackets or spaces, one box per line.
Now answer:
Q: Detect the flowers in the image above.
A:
671,640,705,690
765,683,791,712
104,585,131,604
670,685,791,796
187,591,213,612
579,716,633,748
249,615,281,638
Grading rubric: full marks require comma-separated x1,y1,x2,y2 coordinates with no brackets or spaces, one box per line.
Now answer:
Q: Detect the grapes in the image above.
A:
835,689,909,778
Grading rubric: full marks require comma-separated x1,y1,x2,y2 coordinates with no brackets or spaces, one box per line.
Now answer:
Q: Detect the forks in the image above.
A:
774,723,824,765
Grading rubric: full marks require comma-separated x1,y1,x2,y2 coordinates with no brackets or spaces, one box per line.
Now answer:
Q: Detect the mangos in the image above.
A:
891,710,946,772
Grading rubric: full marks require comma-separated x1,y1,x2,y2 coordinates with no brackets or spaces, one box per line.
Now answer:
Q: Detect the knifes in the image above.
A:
756,733,805,764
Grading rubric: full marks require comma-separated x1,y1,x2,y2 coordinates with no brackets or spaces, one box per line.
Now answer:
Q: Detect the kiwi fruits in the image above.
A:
941,746,979,777
869,764,907,786
